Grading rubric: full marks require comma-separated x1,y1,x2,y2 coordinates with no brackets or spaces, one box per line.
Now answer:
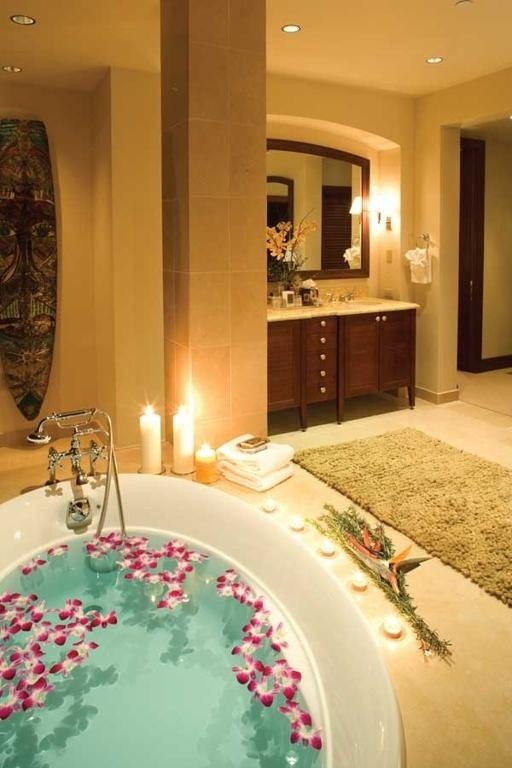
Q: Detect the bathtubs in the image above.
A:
0,472,405,768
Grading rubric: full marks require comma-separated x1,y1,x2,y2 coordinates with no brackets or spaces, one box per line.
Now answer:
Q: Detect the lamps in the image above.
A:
377,187,396,234
348,207,363,226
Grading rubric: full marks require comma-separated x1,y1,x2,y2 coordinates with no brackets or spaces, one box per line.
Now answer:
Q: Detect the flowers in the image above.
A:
265,204,320,270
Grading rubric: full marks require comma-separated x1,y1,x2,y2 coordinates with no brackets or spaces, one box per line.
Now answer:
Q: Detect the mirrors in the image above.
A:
267,139,372,282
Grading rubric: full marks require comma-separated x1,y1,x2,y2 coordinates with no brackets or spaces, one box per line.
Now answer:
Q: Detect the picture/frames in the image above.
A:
280,285,319,309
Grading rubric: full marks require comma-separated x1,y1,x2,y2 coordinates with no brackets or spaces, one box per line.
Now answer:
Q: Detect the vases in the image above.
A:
277,274,305,298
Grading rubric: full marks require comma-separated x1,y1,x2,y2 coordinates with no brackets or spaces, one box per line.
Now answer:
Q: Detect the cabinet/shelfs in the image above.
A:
303,312,345,426
336,307,416,424
267,318,310,433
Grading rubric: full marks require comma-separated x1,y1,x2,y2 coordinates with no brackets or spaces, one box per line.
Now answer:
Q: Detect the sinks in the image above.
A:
354,300,383,306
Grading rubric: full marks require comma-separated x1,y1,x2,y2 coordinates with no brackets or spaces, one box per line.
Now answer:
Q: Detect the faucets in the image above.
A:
71,466,89,487
338,291,354,302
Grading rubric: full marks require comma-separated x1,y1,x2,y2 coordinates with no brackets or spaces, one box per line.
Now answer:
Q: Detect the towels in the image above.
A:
213,434,294,491
403,246,434,286
341,245,362,271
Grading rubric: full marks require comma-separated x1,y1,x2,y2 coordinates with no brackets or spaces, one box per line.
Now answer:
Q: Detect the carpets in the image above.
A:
294,427,511,616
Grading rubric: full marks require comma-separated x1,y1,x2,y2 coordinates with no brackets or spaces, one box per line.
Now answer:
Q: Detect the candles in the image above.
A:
195,442,218,484
139,404,162,475
173,404,194,476
257,490,405,642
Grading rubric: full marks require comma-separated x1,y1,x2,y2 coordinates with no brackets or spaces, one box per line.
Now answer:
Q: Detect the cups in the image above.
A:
272,286,321,310
